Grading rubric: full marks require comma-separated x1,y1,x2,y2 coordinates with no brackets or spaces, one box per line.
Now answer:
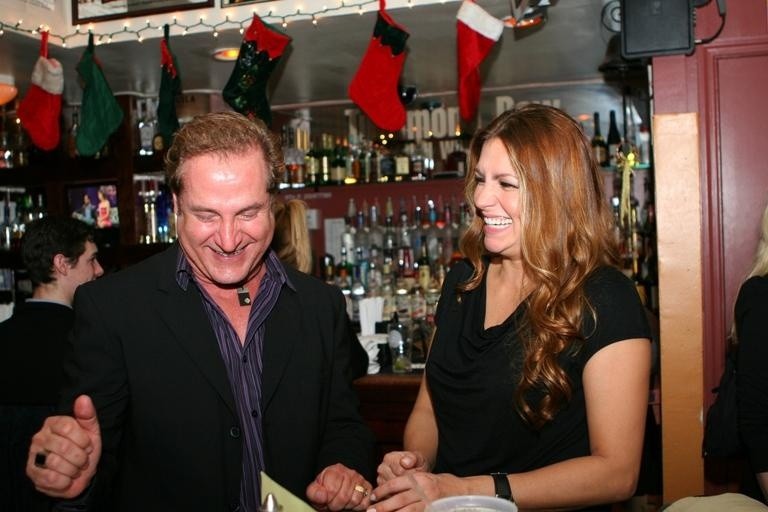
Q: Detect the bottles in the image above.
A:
643,254,658,309
629,203,642,251
638,122,651,165
135,101,154,155
67,109,80,157
627,255,644,305
607,110,622,166
275,126,384,182
320,196,481,321
10,119,29,167
0,189,45,251
643,203,656,251
591,112,607,166
0,130,13,168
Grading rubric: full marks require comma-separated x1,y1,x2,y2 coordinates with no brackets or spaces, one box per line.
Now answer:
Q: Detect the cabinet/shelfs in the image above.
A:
0,96,168,307
276,169,653,454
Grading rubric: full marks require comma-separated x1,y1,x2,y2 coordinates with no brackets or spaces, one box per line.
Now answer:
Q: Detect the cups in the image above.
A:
387,323,414,374
424,495,519,512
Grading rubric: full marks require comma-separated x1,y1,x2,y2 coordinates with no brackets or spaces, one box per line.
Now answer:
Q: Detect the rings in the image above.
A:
34,450,51,469
354,483,368,497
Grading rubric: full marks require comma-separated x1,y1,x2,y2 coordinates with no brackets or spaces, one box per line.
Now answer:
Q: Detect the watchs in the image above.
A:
490,472,515,505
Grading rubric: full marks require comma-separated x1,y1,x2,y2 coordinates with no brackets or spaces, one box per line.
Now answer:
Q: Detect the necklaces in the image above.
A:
197,261,265,307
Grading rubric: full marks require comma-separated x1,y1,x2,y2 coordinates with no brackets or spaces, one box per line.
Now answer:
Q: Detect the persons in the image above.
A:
0,216,105,508
150,134,168,163
25,110,374,512
366,105,653,512
702,208,768,507
